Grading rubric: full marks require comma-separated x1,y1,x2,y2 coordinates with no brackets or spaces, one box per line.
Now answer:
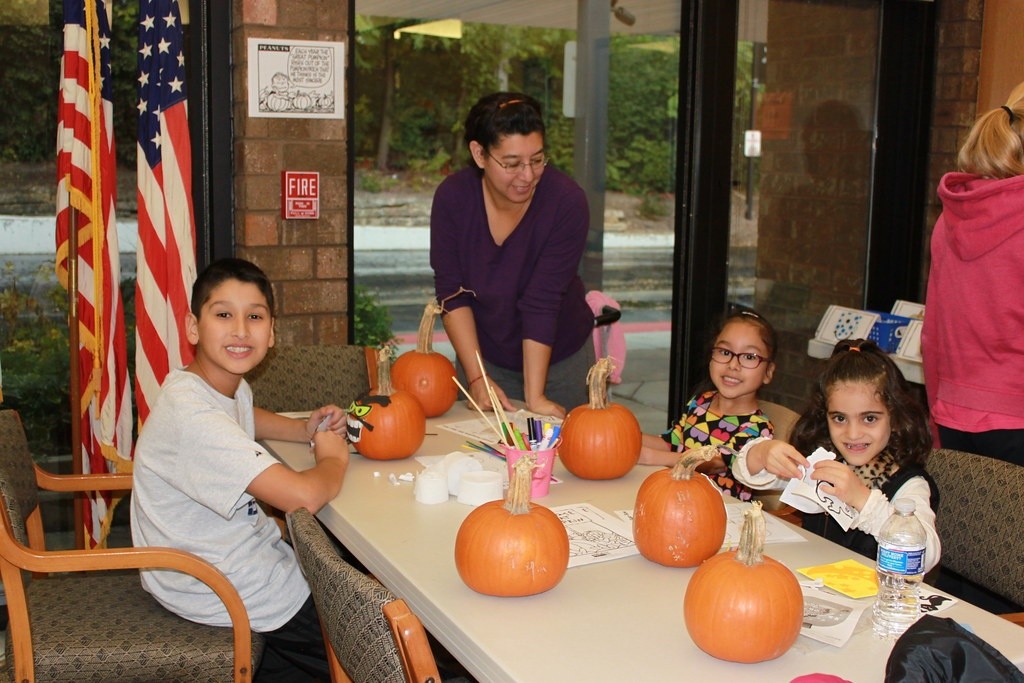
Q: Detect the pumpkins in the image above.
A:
558,358,642,480
391,299,459,418
633,445,728,568
683,502,804,664
456,454,569,598
346,346,426,460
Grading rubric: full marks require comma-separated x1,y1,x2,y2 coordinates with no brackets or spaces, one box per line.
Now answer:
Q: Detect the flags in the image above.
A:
133,0,195,442
53,1,133,551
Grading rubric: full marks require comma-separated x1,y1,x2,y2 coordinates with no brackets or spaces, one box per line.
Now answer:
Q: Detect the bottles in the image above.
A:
872,498,927,643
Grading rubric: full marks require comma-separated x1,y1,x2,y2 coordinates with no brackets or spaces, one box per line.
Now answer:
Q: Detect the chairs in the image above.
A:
753,398,1024,631
237,345,368,417
0,406,290,683
281,507,475,683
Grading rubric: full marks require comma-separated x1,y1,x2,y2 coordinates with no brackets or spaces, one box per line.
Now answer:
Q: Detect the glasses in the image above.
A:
484,150,550,174
708,347,770,369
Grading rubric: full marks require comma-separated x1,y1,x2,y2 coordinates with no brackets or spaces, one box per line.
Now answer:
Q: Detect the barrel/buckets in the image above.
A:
505,449,555,497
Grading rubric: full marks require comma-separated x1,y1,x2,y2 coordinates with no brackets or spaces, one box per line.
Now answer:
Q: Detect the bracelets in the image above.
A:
468,374,487,390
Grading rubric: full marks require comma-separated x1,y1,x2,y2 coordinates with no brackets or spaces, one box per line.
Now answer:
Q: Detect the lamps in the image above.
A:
610,0,635,27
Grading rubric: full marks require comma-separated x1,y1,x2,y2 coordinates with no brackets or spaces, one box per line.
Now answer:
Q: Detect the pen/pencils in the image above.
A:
501,418,560,450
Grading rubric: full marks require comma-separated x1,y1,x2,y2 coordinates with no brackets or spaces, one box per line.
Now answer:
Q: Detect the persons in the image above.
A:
638,307,775,502
733,337,942,579
920,79,1024,472
428,90,597,417
131,257,350,682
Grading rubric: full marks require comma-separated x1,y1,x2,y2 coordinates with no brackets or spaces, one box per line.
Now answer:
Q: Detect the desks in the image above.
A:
261,390,1024,683
